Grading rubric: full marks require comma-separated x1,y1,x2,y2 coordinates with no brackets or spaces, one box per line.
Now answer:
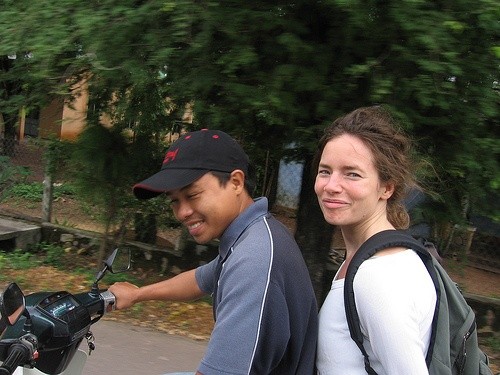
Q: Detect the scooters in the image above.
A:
0,246,131,375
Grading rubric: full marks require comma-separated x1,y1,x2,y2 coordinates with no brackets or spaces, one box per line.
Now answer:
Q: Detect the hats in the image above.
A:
133,127,254,200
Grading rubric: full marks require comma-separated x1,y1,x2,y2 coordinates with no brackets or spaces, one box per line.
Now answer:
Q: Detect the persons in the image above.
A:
106,126,319,375
314,105,438,375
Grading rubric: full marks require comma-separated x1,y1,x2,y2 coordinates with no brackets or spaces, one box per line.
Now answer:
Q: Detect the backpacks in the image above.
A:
343,227,496,375
